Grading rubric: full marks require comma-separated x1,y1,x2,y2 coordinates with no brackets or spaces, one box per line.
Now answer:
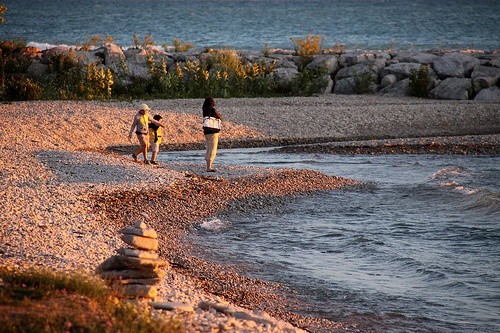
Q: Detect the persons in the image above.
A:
148,115,163,164
202,98,222,172
128,104,164,165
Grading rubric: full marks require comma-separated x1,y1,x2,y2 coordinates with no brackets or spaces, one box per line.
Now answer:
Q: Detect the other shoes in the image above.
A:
208,168,218,172
132,153,138,161
142,160,150,164
150,160,159,164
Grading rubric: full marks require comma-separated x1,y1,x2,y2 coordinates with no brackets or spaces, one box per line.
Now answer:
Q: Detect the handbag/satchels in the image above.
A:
203,116,222,129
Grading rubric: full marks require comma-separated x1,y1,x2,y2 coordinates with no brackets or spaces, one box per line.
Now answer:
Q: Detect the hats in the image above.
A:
138,103,150,110
